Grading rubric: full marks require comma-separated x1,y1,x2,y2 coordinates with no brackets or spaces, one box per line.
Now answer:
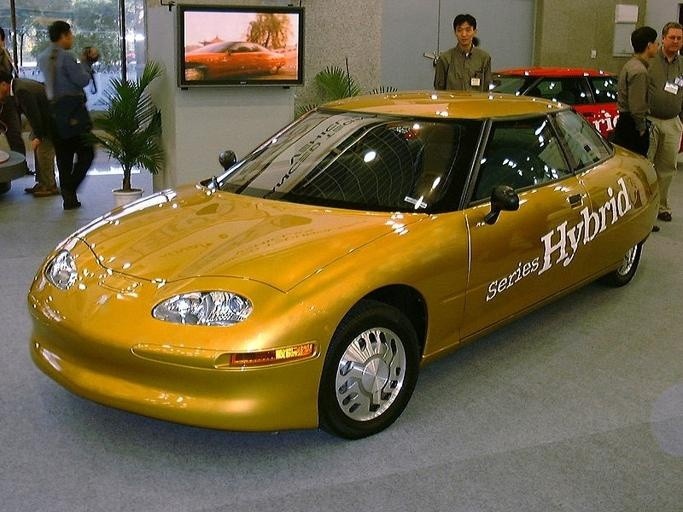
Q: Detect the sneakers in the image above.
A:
657,212,672,221
652,225,660,232
24,184,40,194
33,186,60,197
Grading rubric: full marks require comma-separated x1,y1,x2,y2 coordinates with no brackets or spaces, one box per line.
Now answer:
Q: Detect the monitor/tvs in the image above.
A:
173,4,307,92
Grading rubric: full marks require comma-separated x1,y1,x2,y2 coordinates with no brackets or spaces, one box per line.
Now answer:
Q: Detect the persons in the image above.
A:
37,21,101,210
1,26,38,175
614,27,661,232
1,73,58,196
645,22,683,221
433,14,494,92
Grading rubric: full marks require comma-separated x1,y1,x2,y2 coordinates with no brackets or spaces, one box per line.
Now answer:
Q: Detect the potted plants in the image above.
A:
80,56,168,207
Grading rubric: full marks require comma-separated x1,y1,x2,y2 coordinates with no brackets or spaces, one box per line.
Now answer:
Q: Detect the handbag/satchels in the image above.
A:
46,95,93,146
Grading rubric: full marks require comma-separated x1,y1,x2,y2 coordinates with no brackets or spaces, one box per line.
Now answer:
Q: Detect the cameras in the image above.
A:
84,45,100,66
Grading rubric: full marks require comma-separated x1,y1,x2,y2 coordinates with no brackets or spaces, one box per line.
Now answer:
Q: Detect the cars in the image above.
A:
180,40,286,77
488,66,619,139
27,89,658,439
